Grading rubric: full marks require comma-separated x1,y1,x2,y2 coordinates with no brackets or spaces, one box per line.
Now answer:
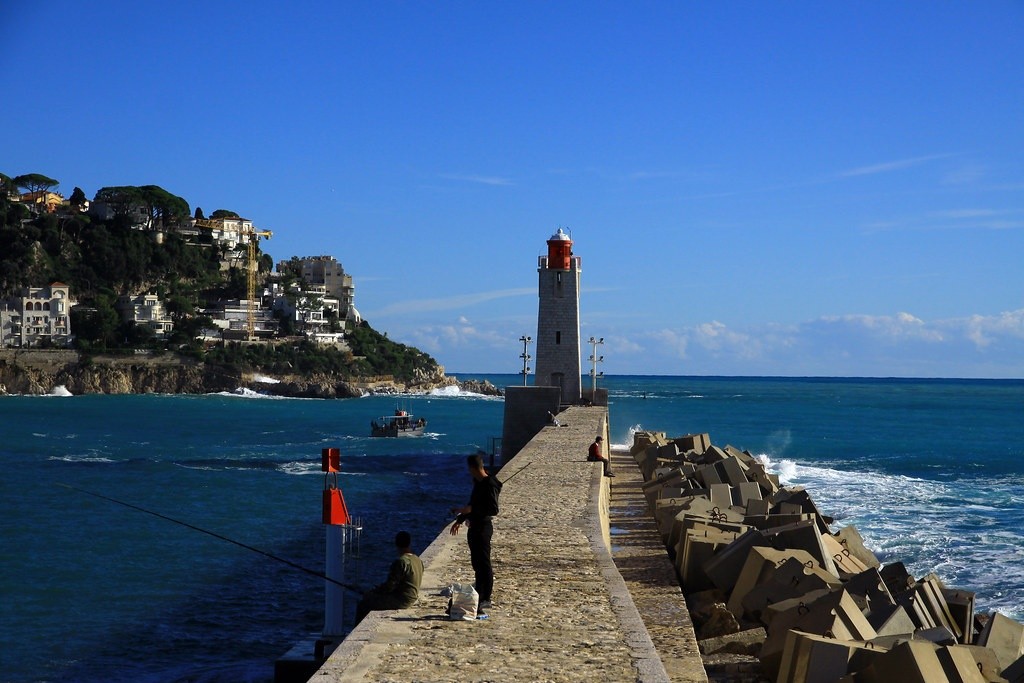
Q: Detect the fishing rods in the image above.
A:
51,480,370,597
443,459,535,528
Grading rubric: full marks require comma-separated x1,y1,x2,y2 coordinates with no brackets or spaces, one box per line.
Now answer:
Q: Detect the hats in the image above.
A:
596,436,604,441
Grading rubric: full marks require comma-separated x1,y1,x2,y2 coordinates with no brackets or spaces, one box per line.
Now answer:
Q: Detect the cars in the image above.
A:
572,398,592,407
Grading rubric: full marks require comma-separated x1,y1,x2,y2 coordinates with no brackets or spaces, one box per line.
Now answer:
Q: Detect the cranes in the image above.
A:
196,217,274,341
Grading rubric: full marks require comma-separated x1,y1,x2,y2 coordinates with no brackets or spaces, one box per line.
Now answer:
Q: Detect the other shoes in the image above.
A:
604,472,615,477
479,600,492,608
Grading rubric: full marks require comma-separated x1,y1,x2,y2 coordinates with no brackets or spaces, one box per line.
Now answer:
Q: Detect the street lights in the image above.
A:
519,336,532,386
587,337,604,391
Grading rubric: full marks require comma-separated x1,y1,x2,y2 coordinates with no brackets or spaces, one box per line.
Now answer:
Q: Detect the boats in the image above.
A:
371,408,427,437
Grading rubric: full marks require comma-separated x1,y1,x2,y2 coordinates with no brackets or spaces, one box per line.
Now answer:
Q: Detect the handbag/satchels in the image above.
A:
450,583,479,621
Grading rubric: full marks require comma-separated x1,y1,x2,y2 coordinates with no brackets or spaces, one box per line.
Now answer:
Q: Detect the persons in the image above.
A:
450,455,503,608
545,410,555,426
588,436,615,477
354,531,424,623
